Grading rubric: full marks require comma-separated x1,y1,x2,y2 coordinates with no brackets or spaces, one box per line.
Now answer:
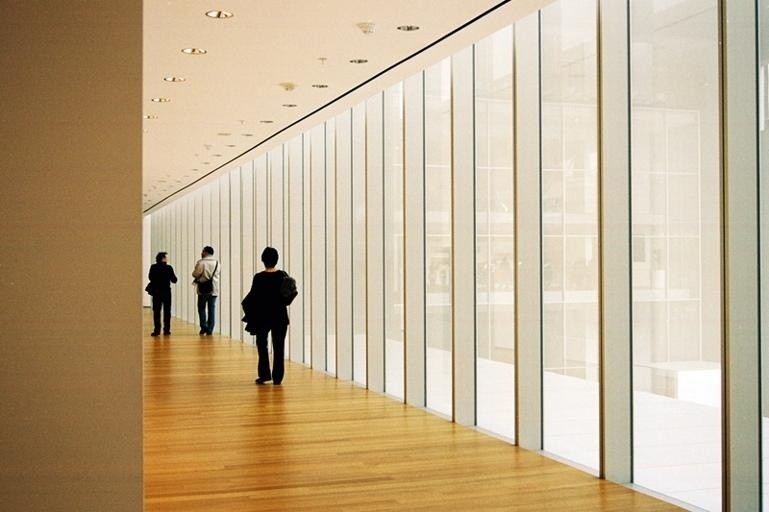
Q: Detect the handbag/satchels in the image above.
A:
281,270,299,307
145,281,159,296
198,278,213,294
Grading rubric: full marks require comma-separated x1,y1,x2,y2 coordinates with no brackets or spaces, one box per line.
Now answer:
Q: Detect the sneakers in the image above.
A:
151,330,171,336
256,376,281,385
200,325,212,335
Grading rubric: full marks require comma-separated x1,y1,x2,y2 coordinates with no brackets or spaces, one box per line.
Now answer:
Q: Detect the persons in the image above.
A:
240,245,299,386
144,251,178,337
192,245,222,338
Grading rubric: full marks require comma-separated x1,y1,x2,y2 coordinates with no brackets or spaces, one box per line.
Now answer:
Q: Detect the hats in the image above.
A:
261,247,279,263
